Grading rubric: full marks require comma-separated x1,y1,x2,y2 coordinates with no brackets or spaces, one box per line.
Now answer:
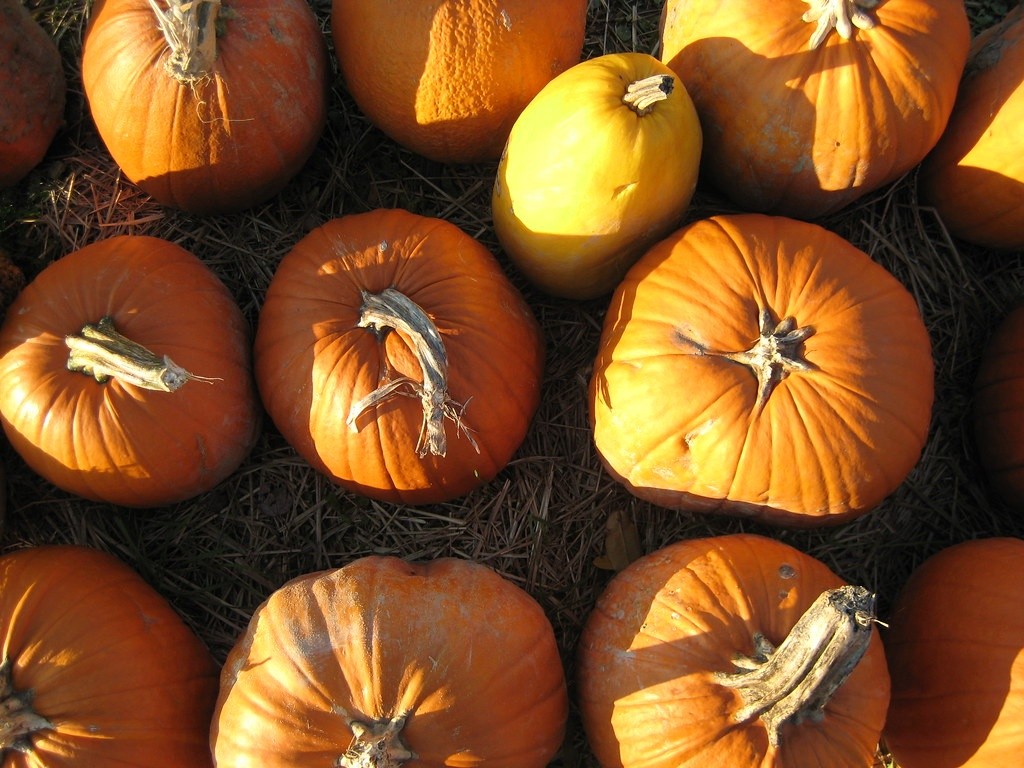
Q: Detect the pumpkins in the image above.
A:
0,0,1024,768
